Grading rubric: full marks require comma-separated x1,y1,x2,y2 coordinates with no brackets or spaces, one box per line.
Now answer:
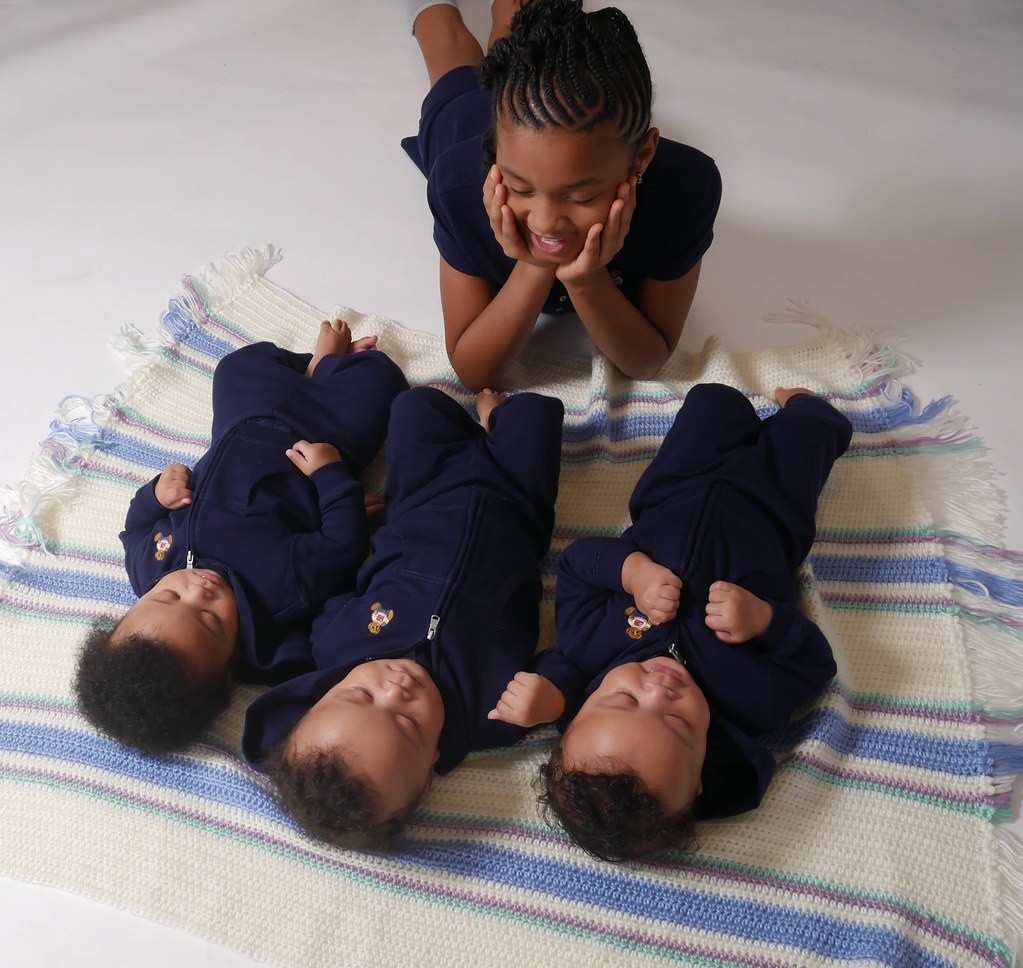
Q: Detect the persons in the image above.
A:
72,318,411,762
242,387,570,848
531,382,853,859
398,1,721,394
